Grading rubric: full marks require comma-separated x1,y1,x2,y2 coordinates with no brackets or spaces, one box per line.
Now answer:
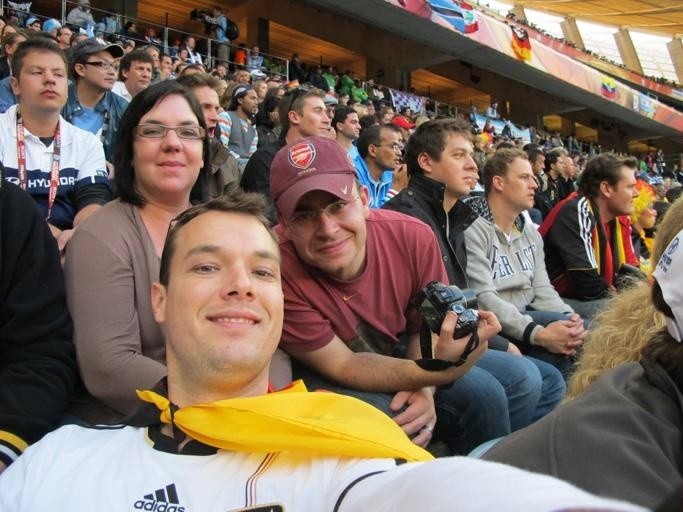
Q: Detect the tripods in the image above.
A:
196,26,217,60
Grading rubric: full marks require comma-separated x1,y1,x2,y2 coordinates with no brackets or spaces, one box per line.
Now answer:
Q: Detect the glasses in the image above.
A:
79,60,116,69
132,125,205,139
376,143,401,153
289,195,361,229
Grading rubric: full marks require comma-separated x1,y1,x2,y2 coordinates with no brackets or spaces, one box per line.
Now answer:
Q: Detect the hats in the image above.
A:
392,116,416,129
44,19,62,31
68,37,124,70
27,18,40,25
269,136,357,224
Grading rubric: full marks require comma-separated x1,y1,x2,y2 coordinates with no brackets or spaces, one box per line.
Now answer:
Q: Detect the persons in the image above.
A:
0,0,682,512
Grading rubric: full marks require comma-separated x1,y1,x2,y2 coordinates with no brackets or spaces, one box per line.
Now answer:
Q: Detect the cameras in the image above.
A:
411,280,480,340
